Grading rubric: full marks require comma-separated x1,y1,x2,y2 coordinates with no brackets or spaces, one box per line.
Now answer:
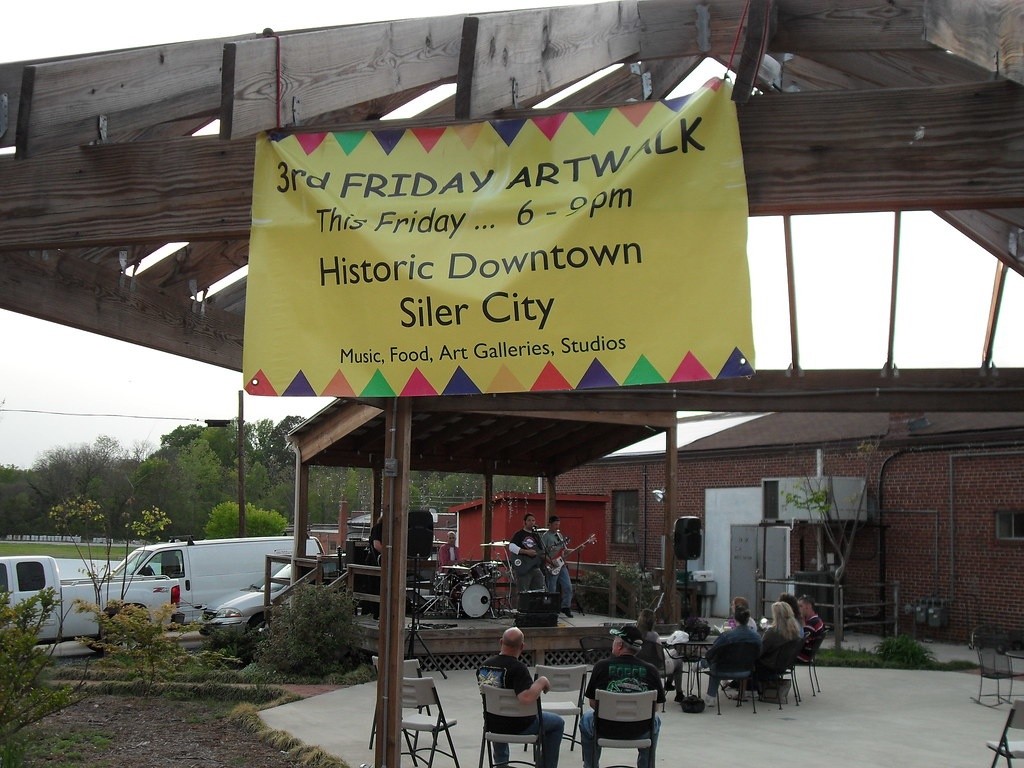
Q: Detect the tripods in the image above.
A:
404,559,458,680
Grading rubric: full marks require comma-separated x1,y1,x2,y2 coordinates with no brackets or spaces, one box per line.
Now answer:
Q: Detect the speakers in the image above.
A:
405,589,434,615
517,591,561,614
407,510,433,559
673,516,702,560
345,541,371,568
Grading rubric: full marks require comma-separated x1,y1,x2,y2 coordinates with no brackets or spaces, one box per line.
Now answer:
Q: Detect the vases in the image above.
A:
680,627,710,637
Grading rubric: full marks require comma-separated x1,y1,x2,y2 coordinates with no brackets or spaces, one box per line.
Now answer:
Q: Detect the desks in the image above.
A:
660,638,721,700
1005,649,1024,659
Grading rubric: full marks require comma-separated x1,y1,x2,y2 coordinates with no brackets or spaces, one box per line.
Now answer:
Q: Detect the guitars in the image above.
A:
511,535,570,577
335,545,343,579
545,533,598,576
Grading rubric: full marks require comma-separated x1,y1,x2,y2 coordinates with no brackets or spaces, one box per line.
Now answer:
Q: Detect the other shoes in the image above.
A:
728,688,739,700
693,661,703,672
664,682,676,691
745,691,759,697
561,607,573,618
674,693,685,702
702,693,716,706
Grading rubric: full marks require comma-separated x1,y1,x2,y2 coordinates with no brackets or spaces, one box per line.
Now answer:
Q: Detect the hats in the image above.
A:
610,626,643,650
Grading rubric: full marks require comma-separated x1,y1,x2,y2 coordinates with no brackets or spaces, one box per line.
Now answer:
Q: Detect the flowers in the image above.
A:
684,616,707,628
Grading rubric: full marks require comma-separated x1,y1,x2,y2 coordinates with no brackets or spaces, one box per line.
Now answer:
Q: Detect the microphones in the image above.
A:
531,525,539,528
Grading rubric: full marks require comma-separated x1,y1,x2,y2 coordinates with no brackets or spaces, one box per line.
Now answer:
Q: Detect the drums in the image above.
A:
470,561,493,583
450,581,492,618
448,572,468,590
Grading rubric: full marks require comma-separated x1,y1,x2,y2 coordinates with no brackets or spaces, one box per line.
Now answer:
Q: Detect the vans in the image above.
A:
213,553,349,645
108,535,326,615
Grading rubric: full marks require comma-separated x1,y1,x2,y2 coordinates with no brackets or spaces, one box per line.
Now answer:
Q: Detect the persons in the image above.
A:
371,514,383,619
795,594,825,665
541,516,575,618
579,625,666,768
476,627,565,768
635,608,686,702
721,601,801,701
779,593,802,622
508,512,557,593
690,607,763,707
728,596,758,633
438,530,462,592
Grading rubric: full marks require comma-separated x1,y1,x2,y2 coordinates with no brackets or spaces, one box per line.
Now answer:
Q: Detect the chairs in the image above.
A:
968,624,1017,709
523,664,589,751
161,556,181,576
580,629,829,717
986,700,1024,768
404,677,460,768
477,685,547,768
368,656,437,750
585,690,655,768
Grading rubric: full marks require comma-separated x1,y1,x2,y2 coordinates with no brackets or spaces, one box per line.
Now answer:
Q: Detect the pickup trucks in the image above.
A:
0,555,182,654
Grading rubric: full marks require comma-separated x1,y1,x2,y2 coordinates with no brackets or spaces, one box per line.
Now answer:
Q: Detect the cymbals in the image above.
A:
478,541,510,547
441,565,469,570
